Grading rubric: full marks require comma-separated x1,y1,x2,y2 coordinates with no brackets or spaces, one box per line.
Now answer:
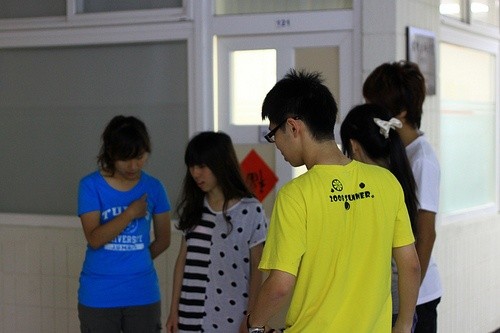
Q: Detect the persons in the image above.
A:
363,61,442,333
165,131,268,333
246,69,420,333
340,104,420,333
78,115,172,333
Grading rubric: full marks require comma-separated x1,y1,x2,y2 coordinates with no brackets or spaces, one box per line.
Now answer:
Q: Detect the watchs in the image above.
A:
246,314,264,333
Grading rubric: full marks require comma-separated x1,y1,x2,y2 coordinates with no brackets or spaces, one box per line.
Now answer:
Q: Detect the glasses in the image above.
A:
265,116,302,143
